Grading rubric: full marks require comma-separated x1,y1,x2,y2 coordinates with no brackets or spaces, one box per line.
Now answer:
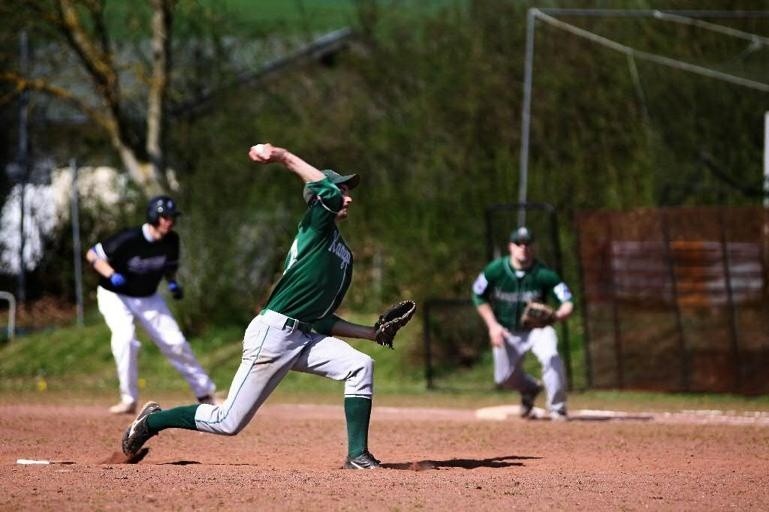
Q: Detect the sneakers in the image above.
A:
121,401,161,457
110,401,136,413
520,379,543,416
346,452,384,471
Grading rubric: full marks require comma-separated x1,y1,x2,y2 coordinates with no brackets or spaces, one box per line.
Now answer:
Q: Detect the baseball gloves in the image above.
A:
520,301,554,330
375,298,415,343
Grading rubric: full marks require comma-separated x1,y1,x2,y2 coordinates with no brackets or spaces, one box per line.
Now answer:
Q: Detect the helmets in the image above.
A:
147,194,180,227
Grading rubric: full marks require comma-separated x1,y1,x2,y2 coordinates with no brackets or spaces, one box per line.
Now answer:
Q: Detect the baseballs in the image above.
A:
253,144,266,156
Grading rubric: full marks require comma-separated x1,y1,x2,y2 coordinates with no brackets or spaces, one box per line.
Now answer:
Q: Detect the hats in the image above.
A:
510,227,535,241
303,169,358,204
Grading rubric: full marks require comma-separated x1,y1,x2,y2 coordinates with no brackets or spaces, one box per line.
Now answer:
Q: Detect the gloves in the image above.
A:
169,282,184,300
111,273,126,285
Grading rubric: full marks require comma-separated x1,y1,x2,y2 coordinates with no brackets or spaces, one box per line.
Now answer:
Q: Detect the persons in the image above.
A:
85,196,218,414
121,143,416,470
470,226,576,422
